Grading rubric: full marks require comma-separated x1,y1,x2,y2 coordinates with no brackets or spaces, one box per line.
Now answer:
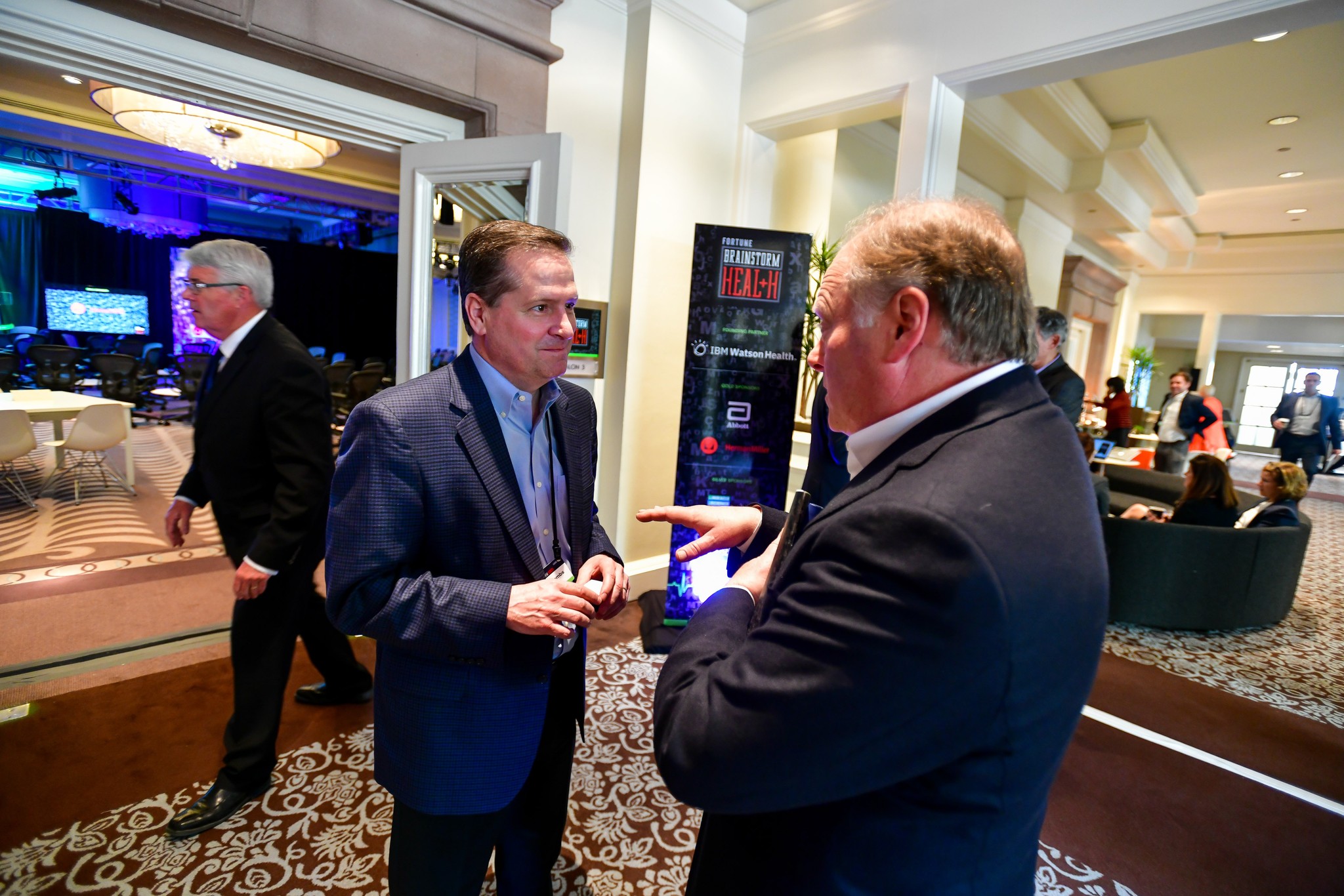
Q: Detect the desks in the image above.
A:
0,390,135,485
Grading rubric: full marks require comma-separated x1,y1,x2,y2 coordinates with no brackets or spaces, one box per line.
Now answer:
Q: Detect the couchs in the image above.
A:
1087,465,1313,632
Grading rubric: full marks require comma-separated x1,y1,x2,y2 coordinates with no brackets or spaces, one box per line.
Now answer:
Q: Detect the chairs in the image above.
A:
315,357,394,438
0,330,213,510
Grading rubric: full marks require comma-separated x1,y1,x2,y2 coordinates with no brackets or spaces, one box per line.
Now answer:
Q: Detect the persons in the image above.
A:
637,200,1110,896
1188,385,1234,461
1226,461,1308,528
164,239,373,841
1077,433,1110,516
1153,371,1217,475
1270,372,1342,486
802,375,849,508
1104,376,1132,447
1121,453,1240,527
1031,306,1086,426
324,221,631,896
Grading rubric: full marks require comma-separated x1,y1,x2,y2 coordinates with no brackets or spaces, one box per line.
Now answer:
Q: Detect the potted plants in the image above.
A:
795,237,846,431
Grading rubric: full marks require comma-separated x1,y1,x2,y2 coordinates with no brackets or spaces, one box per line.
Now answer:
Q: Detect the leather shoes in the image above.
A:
295,682,373,708
166,780,273,838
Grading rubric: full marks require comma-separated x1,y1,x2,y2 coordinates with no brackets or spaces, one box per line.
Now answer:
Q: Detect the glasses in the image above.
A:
176,277,246,296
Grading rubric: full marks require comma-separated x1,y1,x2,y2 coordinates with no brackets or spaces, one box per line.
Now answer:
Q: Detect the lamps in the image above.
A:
34,169,77,199
90,80,344,170
115,181,140,215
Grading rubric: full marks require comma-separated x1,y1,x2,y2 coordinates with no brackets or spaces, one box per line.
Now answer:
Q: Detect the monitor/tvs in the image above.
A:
42,283,151,340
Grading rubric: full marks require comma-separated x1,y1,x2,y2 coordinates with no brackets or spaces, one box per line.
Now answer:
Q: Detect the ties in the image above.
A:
205,345,222,397
